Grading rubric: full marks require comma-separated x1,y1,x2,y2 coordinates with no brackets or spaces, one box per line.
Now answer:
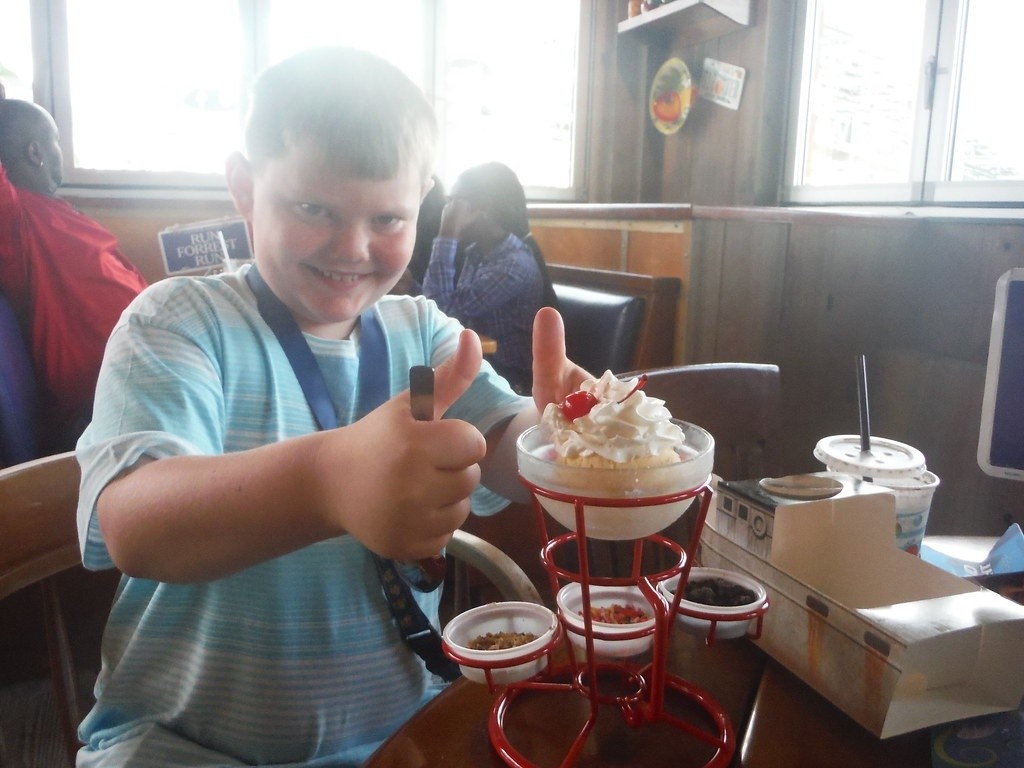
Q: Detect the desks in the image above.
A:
360,522,1024,768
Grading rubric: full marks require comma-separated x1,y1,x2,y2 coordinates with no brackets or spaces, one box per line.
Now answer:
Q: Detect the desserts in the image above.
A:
517,368,713,540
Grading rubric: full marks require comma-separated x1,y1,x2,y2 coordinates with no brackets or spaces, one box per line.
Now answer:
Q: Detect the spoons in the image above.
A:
393,365,449,592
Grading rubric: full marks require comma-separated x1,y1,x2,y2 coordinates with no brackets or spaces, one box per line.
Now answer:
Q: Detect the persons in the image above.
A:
390,160,563,397
0,83,148,449
74,44,597,768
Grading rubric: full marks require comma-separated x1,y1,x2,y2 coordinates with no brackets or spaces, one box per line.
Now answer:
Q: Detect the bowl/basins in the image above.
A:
518,412,715,543
442,602,558,687
556,579,670,658
659,566,768,640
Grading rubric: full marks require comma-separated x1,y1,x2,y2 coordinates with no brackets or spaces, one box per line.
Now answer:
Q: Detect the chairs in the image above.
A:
0,362,780,768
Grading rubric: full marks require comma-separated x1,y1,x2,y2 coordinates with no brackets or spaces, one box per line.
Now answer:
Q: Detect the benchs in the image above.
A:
545,264,681,380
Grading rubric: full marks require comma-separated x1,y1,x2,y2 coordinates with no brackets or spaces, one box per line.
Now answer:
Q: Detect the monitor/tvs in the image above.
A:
978,266,1024,482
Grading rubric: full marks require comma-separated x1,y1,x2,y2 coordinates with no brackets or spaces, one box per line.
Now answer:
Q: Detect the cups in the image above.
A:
827,463,940,560
